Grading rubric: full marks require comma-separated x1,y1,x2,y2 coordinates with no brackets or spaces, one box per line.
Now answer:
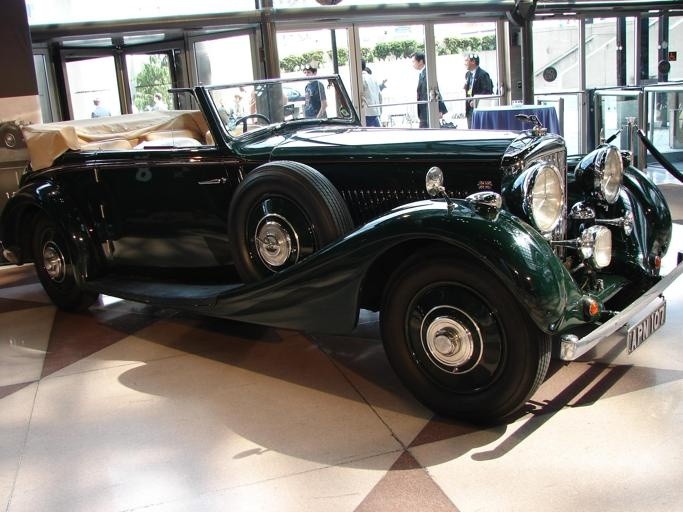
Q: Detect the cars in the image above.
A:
280,85,306,103
0,116,39,149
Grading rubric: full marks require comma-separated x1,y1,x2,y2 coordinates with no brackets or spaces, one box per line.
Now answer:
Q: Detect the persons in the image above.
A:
302,65,328,119
248,91,257,123
360,59,381,127
462,54,493,129
365,67,382,120
91,98,111,119
410,51,449,127
153,93,168,110
233,94,243,117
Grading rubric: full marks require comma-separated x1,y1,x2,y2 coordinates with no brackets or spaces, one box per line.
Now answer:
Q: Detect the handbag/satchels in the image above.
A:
439,122,457,128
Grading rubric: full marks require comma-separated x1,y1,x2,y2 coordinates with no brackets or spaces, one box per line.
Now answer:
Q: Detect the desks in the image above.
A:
471,104,559,136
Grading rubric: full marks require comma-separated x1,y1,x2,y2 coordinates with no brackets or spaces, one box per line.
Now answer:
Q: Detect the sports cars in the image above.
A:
0,71,683,434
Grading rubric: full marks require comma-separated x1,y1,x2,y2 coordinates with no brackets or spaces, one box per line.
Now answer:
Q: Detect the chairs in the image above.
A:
77,129,203,153
